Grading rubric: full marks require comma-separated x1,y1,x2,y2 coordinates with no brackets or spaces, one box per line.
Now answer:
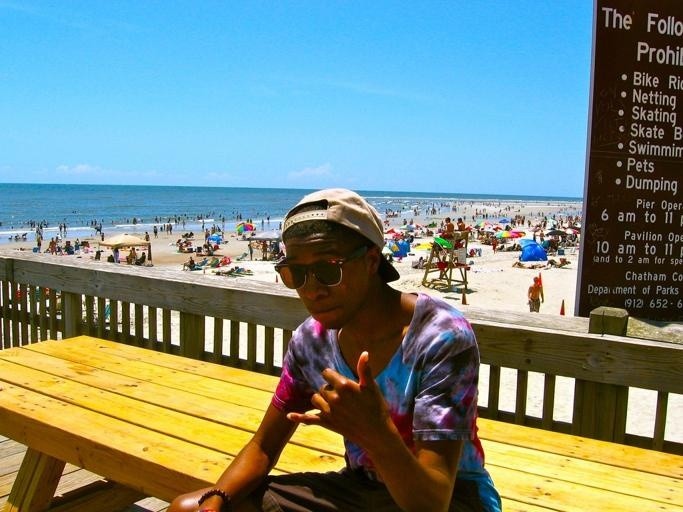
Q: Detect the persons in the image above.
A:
163,188,504,512
0,190,582,271
528,276,544,312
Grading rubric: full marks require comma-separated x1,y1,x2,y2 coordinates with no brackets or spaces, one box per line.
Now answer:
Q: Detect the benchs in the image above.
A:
0,334,683,512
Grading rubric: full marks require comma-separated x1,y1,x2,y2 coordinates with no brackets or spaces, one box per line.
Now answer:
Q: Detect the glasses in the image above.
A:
275,245,372,289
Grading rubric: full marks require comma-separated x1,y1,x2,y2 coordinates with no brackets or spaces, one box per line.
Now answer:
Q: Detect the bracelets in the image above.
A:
197,488,233,505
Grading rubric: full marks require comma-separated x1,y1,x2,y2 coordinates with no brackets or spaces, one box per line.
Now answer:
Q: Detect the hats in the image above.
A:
281,188,400,282
